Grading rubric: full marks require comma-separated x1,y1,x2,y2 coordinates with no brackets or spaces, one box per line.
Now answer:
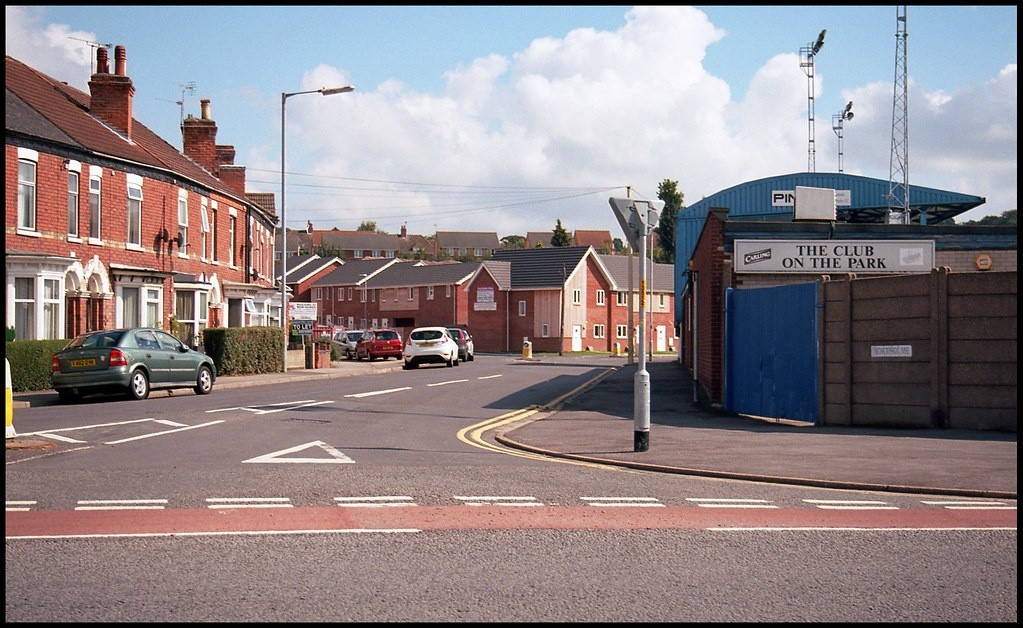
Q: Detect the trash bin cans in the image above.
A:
613,343,621,356
522,341,532,360
6,358,17,439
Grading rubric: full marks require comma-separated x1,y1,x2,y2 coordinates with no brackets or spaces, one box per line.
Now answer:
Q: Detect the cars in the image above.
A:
448,329,474,362
51,328,215,400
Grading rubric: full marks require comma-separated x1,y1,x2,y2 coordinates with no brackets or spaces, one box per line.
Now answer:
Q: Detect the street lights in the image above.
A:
282,86,355,372
359,273,367,329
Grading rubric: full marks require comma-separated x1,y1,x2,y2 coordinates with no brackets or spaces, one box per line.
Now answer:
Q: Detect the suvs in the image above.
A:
404,326,458,368
355,329,403,361
332,329,365,360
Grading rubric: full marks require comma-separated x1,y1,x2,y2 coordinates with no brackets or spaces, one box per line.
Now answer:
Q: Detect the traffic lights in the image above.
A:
609,197,640,252
